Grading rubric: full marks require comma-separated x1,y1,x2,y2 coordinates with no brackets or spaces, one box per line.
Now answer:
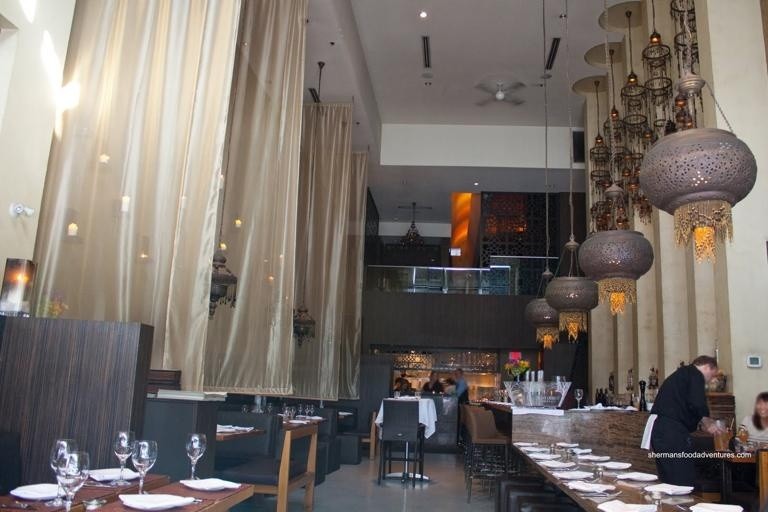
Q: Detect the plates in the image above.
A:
184,484,225,491
10,489,64,501
123,502,175,511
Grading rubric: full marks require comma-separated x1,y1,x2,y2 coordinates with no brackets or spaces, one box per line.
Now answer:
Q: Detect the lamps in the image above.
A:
8,202,23,219
0,257,36,317
496,91,505,101
400,203,425,249
23,207,35,217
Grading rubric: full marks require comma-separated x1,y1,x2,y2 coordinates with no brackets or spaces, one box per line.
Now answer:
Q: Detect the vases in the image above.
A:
511,367,520,377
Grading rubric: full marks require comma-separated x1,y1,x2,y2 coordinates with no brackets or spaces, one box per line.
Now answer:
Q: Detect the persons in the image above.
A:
444,378,456,397
641,355,729,496
740,392,768,449
422,371,444,394
393,378,415,396
454,369,469,404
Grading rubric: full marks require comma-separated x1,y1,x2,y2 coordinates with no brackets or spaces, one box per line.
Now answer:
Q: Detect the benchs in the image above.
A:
314,407,340,473
338,407,358,432
216,409,280,472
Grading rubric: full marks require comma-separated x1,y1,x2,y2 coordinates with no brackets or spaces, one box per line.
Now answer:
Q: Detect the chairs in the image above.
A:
377,399,426,489
222,421,320,512
459,402,508,504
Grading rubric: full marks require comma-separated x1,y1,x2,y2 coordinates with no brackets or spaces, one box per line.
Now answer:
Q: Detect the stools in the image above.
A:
336,434,362,465
503,484,558,512
517,495,578,512
494,475,544,512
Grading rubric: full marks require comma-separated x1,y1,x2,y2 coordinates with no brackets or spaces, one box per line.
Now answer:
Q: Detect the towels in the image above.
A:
690,502,744,512
553,441,579,447
646,482,693,495
234,427,255,431
116,494,194,510
289,420,309,424
89,467,140,482
528,453,561,460
539,461,576,469
551,470,594,479
640,414,658,450
578,454,610,461
568,447,593,454
182,477,242,491
513,442,538,445
339,412,352,415
307,416,322,419
616,472,658,482
216,425,234,432
564,481,615,493
597,461,631,469
16,482,71,500
521,447,548,452
597,500,658,511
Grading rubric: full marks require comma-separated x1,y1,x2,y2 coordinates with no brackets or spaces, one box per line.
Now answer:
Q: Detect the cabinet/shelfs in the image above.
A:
482,400,658,477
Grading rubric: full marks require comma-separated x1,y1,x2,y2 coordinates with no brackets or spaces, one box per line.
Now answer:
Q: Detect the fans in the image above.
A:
474,79,527,107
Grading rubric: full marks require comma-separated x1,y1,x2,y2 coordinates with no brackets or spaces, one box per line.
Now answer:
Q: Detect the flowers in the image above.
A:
503,358,530,372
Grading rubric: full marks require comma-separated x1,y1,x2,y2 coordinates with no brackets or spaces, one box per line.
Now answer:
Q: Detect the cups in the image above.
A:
55,453,90,512
185,432,207,480
46,438,78,509
132,440,158,495
108,431,134,486
574,389,583,410
